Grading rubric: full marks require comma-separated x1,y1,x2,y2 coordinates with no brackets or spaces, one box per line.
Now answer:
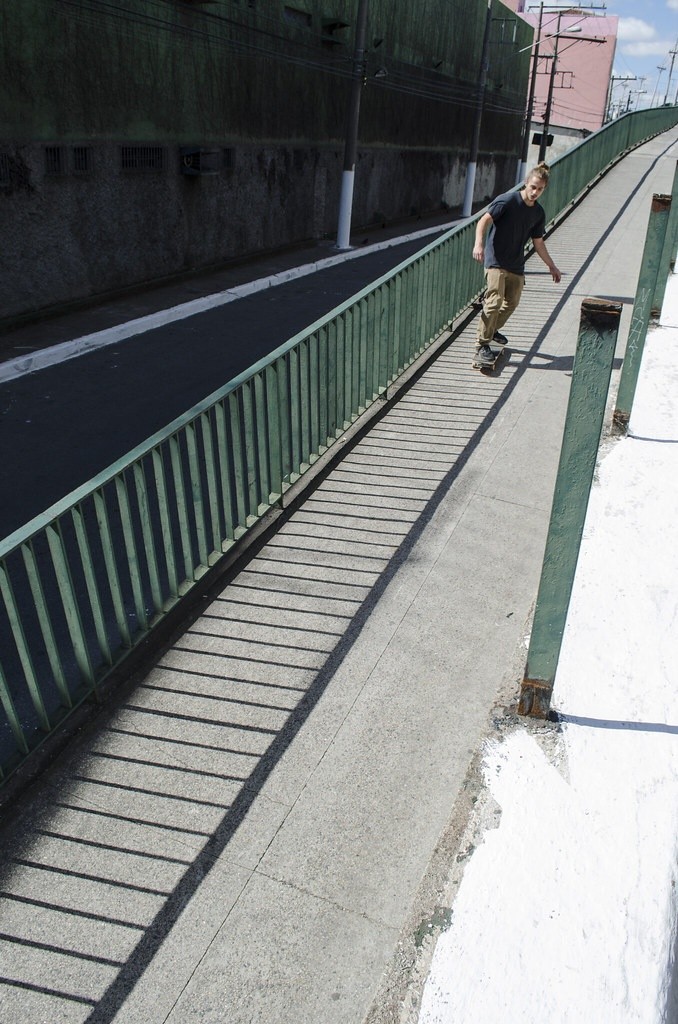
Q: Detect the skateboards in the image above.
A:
470,335,506,371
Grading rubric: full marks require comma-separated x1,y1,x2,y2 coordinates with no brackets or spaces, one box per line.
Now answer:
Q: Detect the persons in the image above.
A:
473,161,561,361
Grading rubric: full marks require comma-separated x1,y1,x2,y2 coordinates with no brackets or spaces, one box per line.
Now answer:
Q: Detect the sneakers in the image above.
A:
476,345,494,360
492,331,508,344
